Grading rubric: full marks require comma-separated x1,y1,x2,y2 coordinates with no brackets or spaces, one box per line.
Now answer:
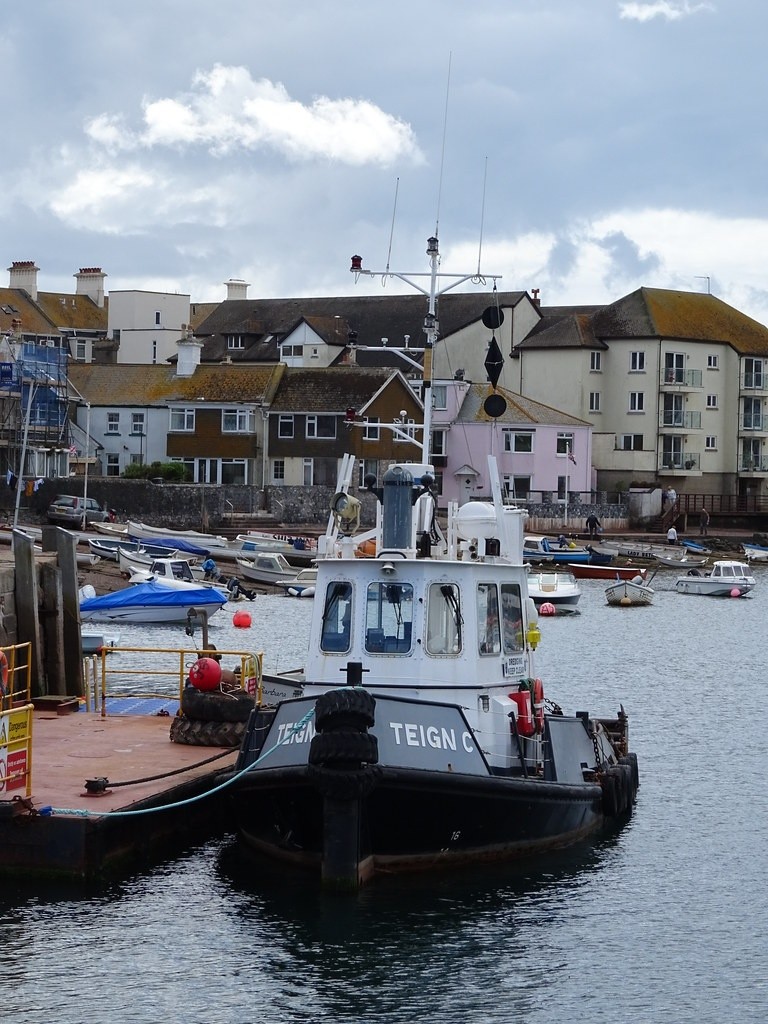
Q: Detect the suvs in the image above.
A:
45,494,112,532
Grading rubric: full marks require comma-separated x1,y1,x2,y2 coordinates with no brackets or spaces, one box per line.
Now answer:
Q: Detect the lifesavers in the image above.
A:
533,678,544,730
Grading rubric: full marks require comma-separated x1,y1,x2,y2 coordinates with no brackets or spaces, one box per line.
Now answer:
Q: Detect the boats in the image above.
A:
30,543,104,569
602,536,692,558
568,558,648,580
523,566,585,616
0,520,50,546
88,518,133,539
236,531,322,561
234,551,305,585
116,545,239,601
672,560,757,598
121,520,226,549
518,534,607,565
652,552,711,568
85,540,180,568
201,39,646,915
741,540,768,563
74,574,230,625
274,565,380,602
603,571,659,606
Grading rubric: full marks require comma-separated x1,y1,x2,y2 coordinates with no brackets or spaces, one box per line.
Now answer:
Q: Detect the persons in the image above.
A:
667,525,678,545
662,489,668,511
586,512,602,541
667,486,677,505
699,507,710,534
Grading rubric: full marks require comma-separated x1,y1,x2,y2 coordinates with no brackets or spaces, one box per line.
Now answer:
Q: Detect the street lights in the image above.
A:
79,401,93,532
